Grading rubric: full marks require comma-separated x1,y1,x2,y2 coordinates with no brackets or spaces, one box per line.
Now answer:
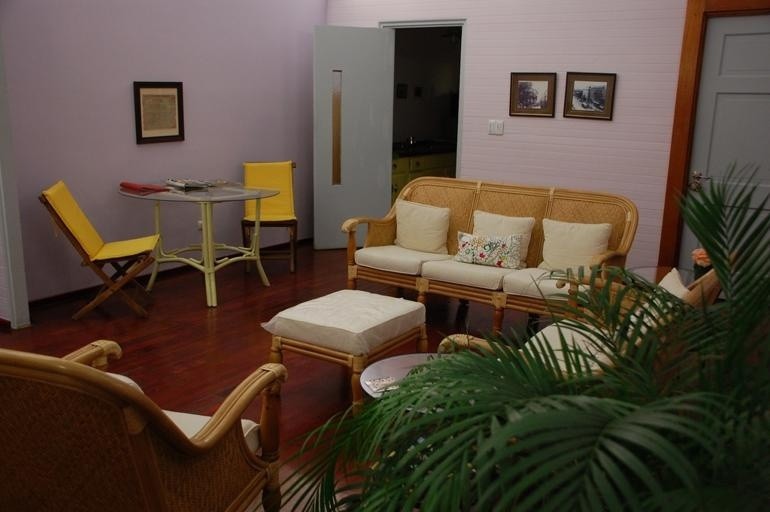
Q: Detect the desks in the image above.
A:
118,178,280,306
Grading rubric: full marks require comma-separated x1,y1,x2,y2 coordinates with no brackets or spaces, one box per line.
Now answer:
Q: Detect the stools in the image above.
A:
261,288,427,463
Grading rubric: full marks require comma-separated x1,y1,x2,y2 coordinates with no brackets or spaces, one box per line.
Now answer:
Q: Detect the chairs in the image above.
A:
1,336,286,512
437,253,730,389
242,159,303,273
38,179,161,320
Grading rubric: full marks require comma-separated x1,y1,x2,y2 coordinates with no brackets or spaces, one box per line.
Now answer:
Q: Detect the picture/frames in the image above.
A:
562,71,617,121
488,119,506,136
134,81,185,146
508,71,558,119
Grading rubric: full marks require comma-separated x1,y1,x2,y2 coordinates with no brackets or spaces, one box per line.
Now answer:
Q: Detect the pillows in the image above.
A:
394,198,450,255
472,207,536,269
539,217,612,289
454,231,522,269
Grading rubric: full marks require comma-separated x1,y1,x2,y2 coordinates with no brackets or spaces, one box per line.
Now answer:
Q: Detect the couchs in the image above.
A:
341,176,639,346
